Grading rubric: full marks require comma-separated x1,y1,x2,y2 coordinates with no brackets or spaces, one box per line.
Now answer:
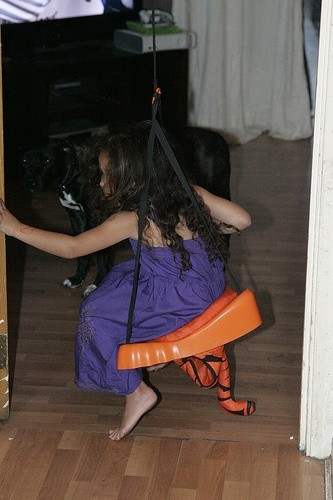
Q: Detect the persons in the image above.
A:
0,132,252,441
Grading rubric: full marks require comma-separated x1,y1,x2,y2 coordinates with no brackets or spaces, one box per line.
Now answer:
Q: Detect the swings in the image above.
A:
116,0,264,369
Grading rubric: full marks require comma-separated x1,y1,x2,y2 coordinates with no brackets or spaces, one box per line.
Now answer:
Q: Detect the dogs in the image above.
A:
20,126,231,300
